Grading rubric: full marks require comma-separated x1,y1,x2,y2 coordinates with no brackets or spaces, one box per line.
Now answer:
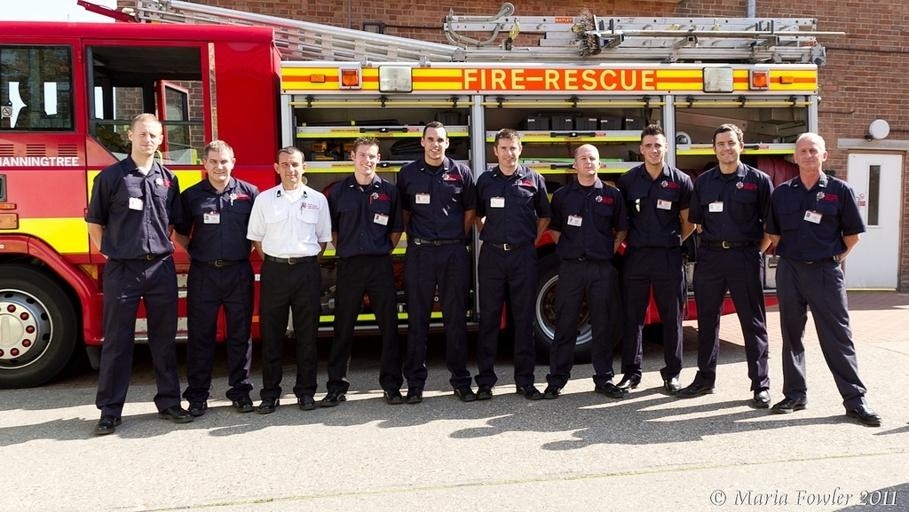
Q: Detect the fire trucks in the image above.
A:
0,1,821,385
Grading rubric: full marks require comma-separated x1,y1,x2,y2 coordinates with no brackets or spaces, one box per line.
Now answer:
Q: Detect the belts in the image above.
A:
492,242,523,251
413,237,460,246
269,256,310,265
206,257,237,266
135,253,163,260
708,240,747,249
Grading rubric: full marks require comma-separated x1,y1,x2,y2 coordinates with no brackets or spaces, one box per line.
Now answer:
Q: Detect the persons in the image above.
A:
615,123,697,395
475,128,551,401
85,112,193,436
396,121,477,404
173,139,260,417
764,131,882,426
319,135,404,407
544,144,628,400
676,123,775,408
245,145,333,414
